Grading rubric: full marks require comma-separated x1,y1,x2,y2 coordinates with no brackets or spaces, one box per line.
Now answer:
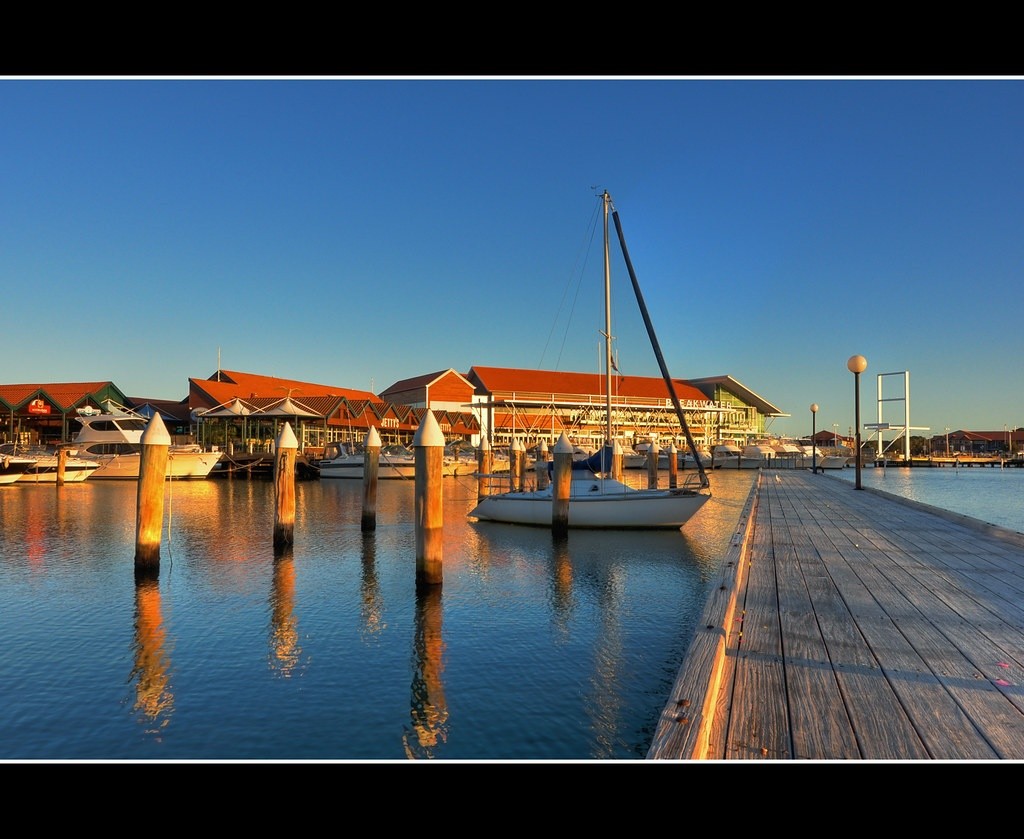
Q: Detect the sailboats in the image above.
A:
462,187,713,530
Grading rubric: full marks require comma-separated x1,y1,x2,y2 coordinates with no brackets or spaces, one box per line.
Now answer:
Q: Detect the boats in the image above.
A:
319,439,849,478
0,413,223,484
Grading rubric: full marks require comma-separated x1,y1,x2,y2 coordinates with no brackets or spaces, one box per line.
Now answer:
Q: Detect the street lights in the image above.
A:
944,427,951,457
847,354,867,490
833,423,839,453
810,403,819,475
